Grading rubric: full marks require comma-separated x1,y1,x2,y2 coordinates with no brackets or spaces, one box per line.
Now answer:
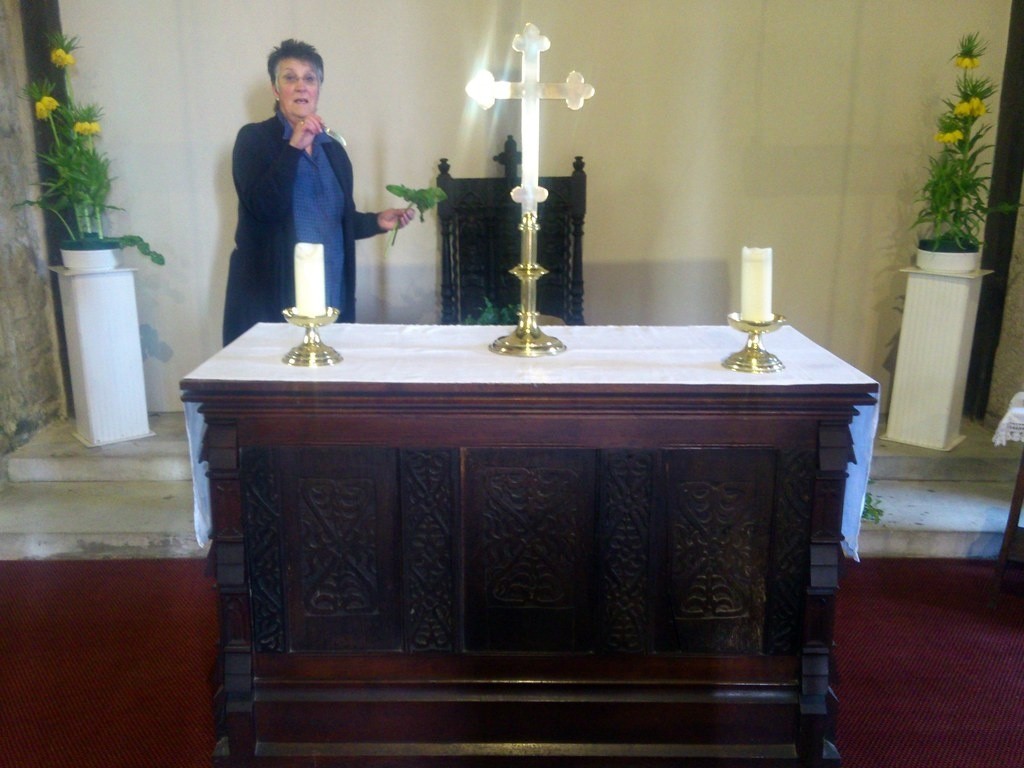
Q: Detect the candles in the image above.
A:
294,241,327,318
741,247,775,324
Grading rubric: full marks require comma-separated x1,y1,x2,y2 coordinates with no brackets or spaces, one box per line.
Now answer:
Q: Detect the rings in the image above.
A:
300,120,304,125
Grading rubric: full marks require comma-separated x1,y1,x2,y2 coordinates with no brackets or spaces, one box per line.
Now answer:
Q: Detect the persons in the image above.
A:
222,38,416,350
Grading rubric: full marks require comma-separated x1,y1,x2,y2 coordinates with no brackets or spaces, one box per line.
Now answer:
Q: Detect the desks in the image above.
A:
173,321,879,767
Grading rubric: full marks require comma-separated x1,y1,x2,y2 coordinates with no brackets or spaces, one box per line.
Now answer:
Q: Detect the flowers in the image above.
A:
908,30,1024,252
9,30,166,270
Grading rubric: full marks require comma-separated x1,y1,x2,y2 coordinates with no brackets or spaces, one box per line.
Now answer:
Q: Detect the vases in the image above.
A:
916,239,980,273
59,240,124,271
